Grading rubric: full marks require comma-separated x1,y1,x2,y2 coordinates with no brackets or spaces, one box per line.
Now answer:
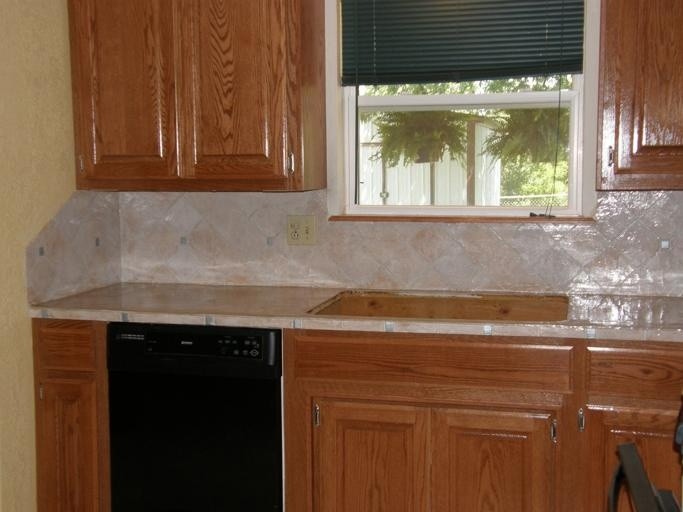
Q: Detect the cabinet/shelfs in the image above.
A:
281,328,574,512
595,0,683,190
67,0,327,192
31,317,112,512
574,338,683,512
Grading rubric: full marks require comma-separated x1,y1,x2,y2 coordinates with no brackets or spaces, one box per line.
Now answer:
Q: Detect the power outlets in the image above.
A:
287,215,317,246
